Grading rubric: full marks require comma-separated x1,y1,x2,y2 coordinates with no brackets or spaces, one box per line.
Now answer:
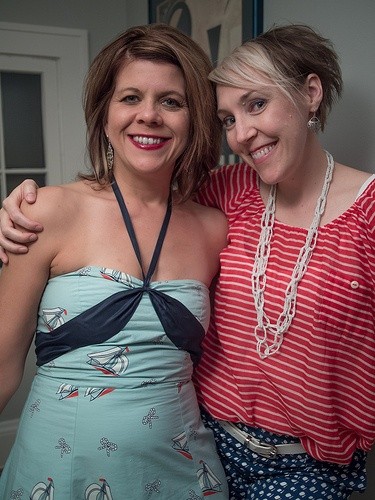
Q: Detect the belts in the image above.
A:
217,420,306,460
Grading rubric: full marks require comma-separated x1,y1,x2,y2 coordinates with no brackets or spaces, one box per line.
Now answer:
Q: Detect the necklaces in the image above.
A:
251,146,334,360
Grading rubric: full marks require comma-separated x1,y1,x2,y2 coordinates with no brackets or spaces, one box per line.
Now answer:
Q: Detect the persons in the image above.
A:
1,21,375,500
1,22,230,500
